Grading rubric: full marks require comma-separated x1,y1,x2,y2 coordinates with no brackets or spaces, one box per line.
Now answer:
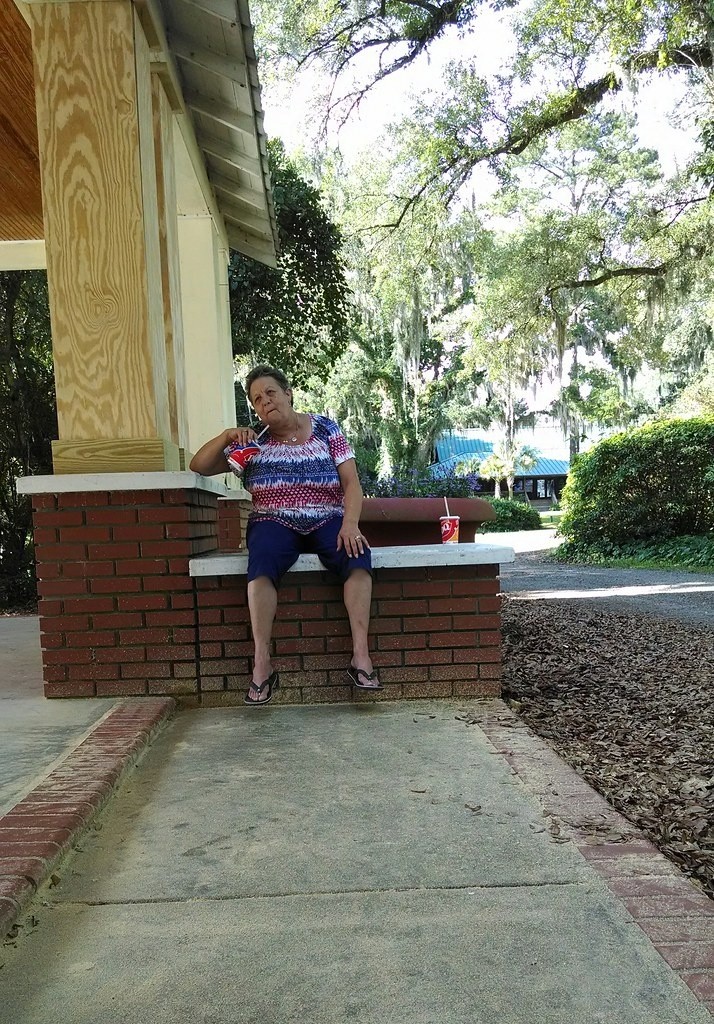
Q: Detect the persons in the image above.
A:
188,364,385,706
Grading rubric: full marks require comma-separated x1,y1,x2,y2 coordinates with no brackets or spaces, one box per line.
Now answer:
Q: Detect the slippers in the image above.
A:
347,664,385,691
242,671,278,705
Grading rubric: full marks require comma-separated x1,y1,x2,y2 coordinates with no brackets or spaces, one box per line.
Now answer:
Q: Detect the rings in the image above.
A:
242,430,247,432
355,536,361,540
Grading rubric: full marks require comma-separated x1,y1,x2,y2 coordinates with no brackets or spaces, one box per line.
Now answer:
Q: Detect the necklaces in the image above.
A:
283,413,299,443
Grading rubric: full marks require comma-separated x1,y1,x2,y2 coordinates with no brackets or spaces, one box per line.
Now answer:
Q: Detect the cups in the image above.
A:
228,440,262,473
439,516,460,544
